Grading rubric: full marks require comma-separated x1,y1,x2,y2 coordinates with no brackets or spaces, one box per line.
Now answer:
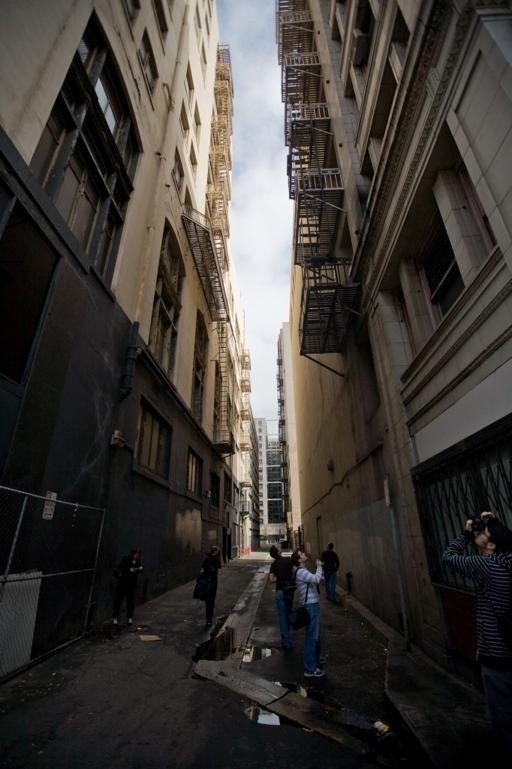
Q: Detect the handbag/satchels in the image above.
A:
288,607,309,631
193,578,210,603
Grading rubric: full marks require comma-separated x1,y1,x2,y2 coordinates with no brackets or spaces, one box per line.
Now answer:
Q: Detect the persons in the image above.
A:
191,544,225,627
438,508,511,768
266,544,298,652
319,542,340,600
110,547,145,626
290,547,327,679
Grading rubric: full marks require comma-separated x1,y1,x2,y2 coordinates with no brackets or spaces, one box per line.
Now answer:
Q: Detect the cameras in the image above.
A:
467,515,489,533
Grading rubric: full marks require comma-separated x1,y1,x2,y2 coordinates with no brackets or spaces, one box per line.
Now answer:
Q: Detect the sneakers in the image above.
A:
304,653,326,677
